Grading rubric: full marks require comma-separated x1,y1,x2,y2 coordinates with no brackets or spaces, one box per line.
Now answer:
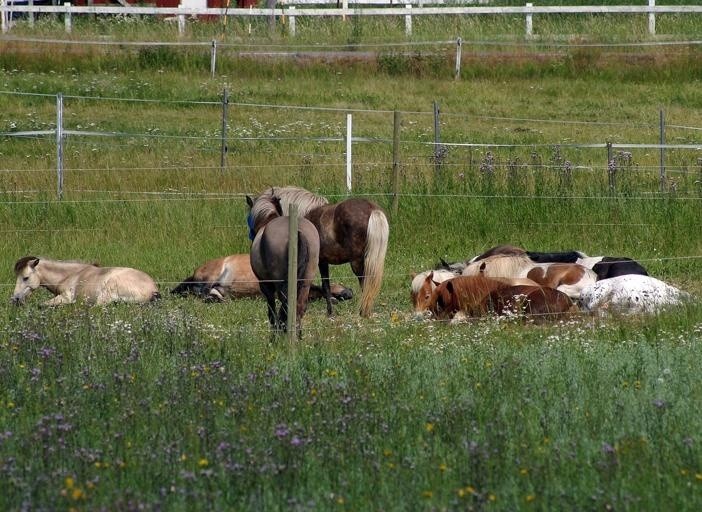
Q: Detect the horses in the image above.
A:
13,256,162,311
265,185,389,319
246,183,320,342
170,253,354,304
410,245,692,328
